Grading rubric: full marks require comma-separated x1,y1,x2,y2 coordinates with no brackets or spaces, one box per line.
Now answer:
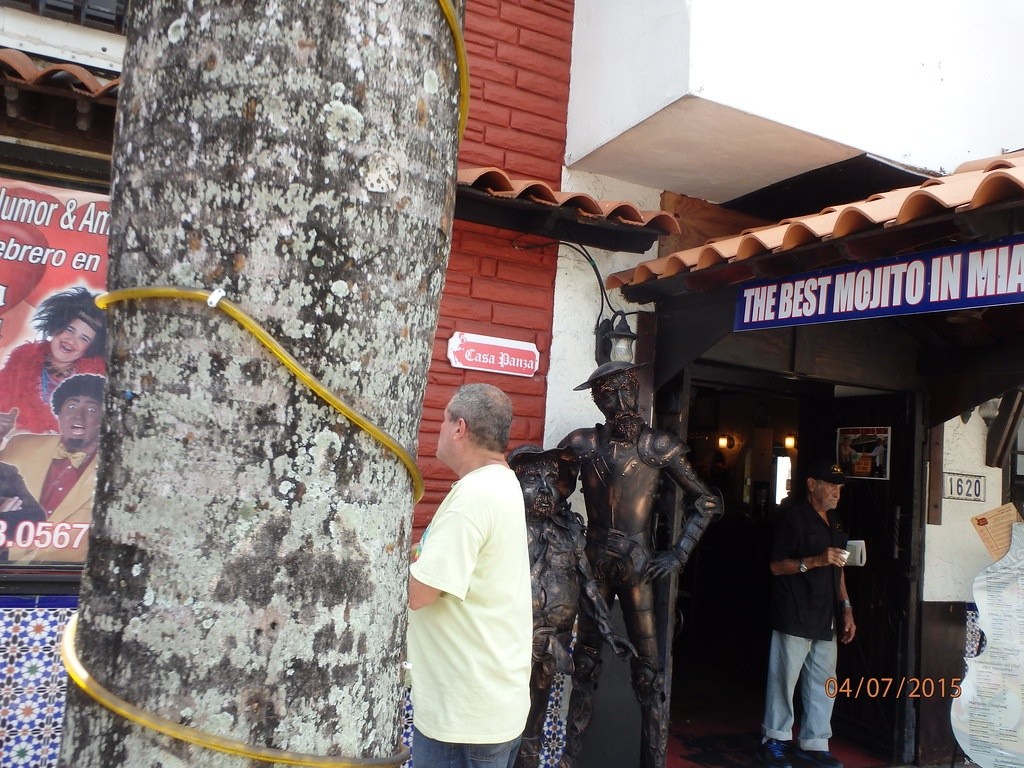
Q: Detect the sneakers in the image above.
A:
753,735,793,768
793,747,844,768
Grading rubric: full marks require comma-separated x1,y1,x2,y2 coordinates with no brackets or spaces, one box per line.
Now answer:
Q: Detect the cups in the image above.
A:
846,540,866,566
839,550,851,567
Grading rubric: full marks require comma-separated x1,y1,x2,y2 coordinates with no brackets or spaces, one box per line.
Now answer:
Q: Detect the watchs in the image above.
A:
800,558,807,573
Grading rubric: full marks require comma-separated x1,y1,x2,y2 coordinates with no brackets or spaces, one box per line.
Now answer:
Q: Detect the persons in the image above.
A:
405,382,532,768
0,286,107,565
840,436,856,476
553,362,722,768
869,435,884,478
759,456,856,768
504,444,639,768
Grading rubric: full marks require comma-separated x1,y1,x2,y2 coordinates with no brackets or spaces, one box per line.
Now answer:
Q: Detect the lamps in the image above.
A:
594,310,638,365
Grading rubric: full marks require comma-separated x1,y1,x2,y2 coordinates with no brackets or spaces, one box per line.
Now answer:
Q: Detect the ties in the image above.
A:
51,443,91,470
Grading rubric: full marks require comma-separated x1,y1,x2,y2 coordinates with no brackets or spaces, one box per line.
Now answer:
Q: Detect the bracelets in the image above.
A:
839,599,850,609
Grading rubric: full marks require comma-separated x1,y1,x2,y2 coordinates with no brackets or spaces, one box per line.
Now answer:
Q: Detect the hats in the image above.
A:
801,459,847,484
